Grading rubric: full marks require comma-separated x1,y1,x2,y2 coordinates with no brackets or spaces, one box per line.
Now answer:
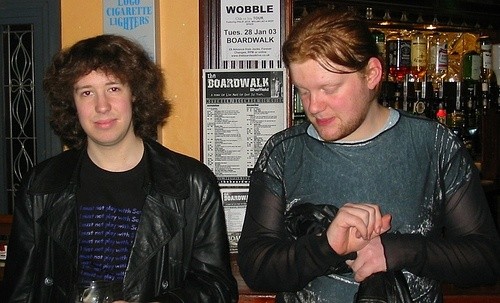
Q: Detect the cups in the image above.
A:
76,280,114,303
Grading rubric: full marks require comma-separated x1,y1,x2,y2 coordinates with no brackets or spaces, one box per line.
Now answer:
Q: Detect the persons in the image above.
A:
237,8,500,303
0,34,238,303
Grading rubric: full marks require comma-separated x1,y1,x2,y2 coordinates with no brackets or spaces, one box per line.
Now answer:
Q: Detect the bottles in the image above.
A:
372,29,492,140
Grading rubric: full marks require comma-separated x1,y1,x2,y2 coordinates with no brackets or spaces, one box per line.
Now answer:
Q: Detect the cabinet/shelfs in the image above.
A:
284,0,500,193
